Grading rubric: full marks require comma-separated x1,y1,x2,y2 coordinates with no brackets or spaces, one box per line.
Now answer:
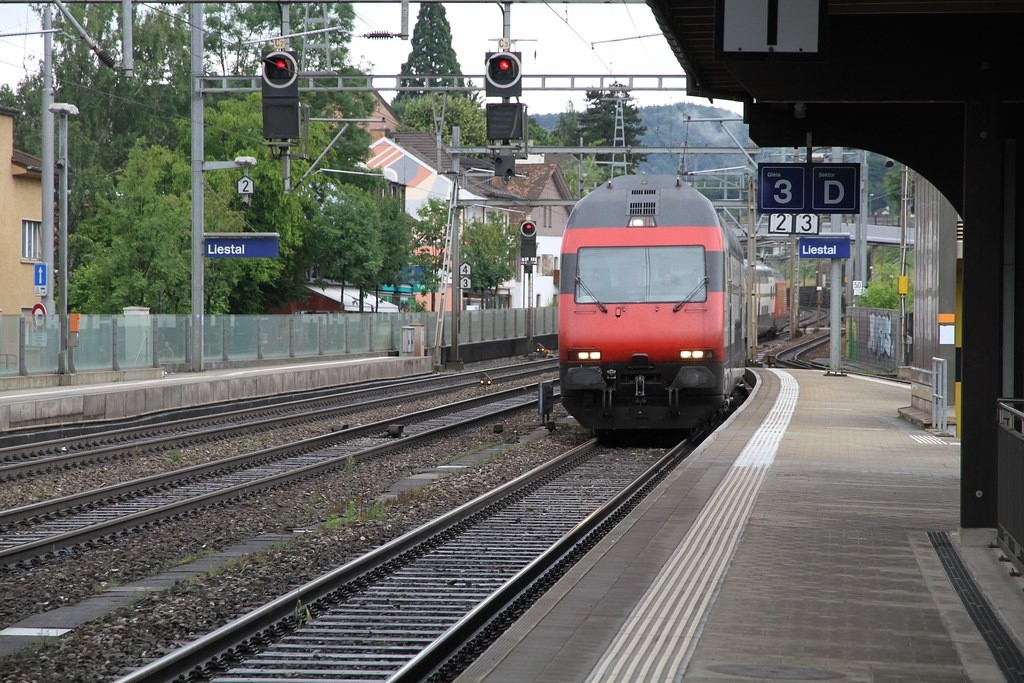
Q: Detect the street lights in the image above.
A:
49,101,78,374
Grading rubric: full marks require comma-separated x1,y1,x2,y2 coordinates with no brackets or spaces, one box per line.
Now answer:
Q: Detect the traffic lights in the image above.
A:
263,56,293,81
490,56,516,81
521,222,537,237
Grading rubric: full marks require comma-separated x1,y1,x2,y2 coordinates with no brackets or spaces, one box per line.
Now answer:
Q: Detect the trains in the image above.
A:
558,170,788,434
